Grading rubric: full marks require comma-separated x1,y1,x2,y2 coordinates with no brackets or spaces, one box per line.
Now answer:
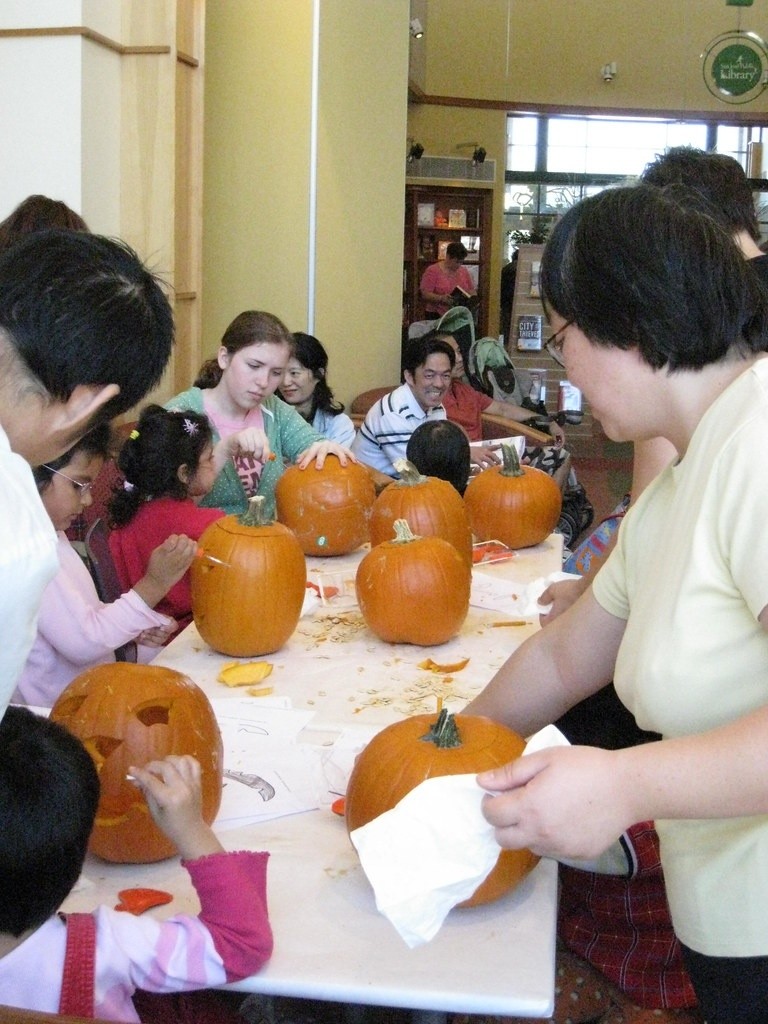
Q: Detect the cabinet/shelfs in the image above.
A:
401,157,503,383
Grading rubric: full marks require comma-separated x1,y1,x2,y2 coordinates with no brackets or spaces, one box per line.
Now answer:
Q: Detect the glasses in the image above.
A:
42,464,98,497
542,318,573,368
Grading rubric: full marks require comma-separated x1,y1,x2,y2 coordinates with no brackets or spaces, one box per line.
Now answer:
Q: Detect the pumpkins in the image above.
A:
347,710,544,905
274,446,560,648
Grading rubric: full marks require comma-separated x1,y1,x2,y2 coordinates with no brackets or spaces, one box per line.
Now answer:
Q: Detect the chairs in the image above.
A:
84,422,137,663
349,386,552,500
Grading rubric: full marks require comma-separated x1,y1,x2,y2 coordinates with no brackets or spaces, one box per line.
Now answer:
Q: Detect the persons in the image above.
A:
406,420,470,498
355,340,503,483
10,425,204,724
453,188,768,1022
420,242,477,321
0,228,175,723
538,145,768,628
107,405,229,649
272,332,357,465
1,705,272,1024
164,311,359,523
422,329,565,450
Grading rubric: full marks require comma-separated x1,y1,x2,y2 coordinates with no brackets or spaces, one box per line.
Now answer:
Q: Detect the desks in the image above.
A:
60,525,565,1017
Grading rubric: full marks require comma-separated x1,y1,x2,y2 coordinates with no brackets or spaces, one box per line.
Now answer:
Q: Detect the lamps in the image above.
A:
601,61,617,83
411,19,424,39
